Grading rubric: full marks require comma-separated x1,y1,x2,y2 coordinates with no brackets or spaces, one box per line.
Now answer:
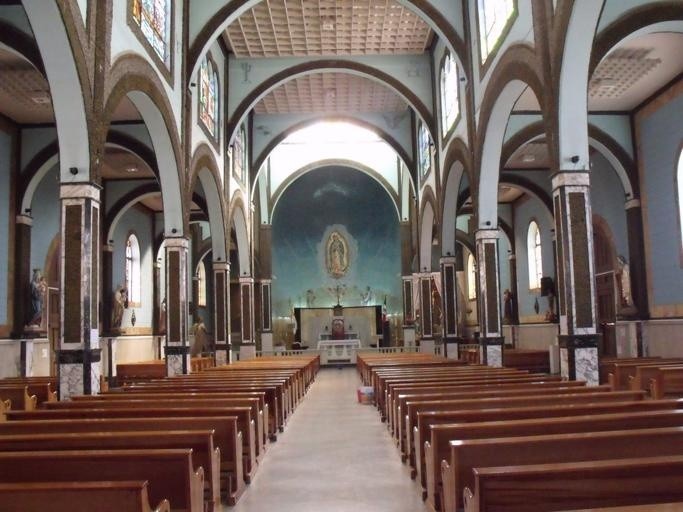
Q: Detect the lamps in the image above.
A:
596,79,615,92
520,155,536,163
124,164,139,174
321,18,336,33
326,87,336,100
28,91,53,104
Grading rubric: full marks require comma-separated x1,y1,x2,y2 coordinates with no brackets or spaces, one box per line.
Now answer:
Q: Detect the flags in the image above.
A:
382,296,386,324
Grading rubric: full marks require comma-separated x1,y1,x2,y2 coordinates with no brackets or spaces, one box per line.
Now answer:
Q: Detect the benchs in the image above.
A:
0,353,321,511
356,351,683,512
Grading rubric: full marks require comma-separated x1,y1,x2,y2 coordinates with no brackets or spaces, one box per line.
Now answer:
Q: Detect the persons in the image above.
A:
359,285,372,306
192,314,207,358
36,275,46,298
110,282,129,330
614,253,632,307
326,233,346,274
304,289,316,308
500,289,512,324
26,266,47,328
